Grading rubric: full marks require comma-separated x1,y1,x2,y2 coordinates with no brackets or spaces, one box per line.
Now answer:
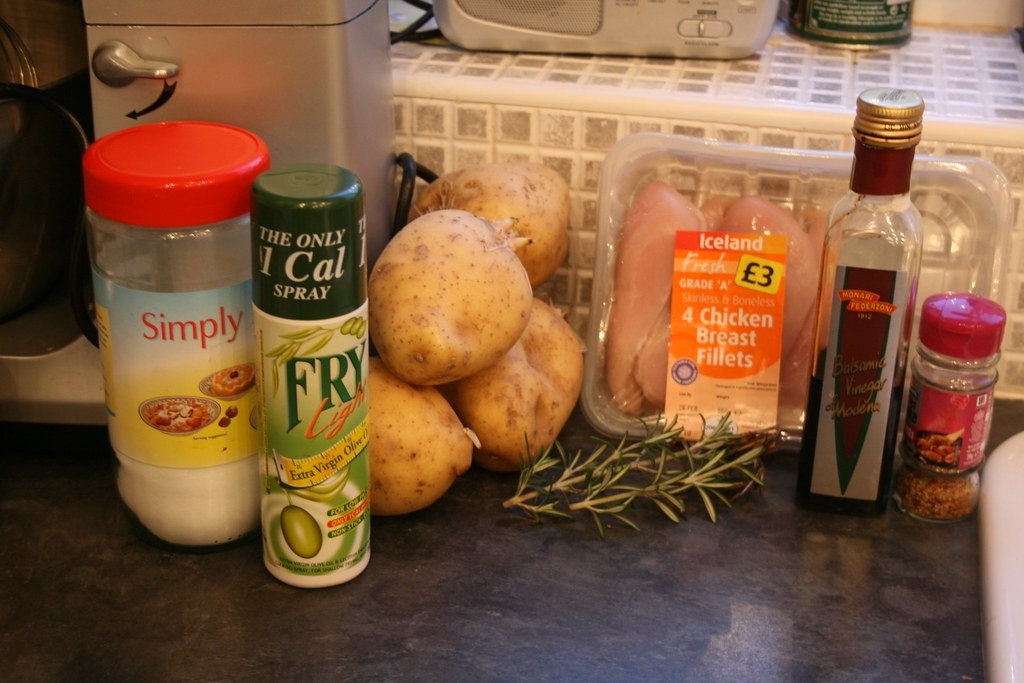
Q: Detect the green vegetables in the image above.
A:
503,412,780,535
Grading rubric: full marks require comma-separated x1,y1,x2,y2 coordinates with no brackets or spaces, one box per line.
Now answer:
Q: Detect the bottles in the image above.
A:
893,289,1008,527
76,119,376,592
795,84,926,520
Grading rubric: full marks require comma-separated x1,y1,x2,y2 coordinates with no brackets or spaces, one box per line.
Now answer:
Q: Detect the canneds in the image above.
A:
79,119,271,547
787,0,914,50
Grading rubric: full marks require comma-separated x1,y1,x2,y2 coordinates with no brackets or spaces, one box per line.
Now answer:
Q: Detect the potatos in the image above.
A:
368,162,584,515
